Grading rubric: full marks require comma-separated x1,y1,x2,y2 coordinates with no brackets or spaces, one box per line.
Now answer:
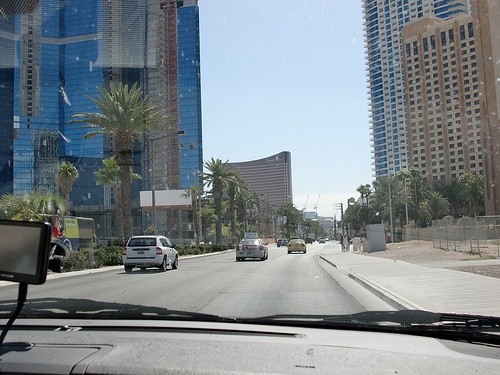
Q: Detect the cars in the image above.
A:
316,236,329,243
287,239,306,254
277,238,289,247
305,238,312,244
236,238,269,261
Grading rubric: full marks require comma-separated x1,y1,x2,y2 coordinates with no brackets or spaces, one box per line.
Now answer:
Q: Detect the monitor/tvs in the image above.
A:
0,219,52,285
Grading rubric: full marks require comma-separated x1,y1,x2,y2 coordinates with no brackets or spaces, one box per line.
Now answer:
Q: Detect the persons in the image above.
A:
387,233,392,243
340,233,351,252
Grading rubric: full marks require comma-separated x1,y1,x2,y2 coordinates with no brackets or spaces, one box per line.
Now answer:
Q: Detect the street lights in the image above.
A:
149,129,187,235
243,193,264,231
404,177,412,225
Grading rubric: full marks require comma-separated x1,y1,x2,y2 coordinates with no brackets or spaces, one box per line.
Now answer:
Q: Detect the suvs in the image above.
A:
121,234,178,272
